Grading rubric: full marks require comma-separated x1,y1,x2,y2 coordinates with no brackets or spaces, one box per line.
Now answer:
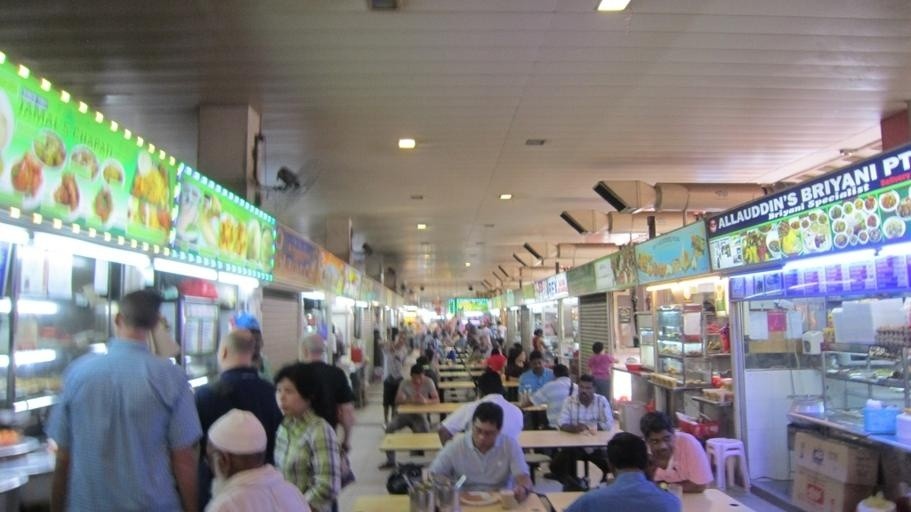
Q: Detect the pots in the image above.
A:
787,393,833,426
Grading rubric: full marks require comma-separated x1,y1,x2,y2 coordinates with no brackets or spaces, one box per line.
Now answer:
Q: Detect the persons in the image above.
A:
229,314,272,382
41,289,204,511
272,361,342,512
193,325,284,511
274,331,357,454
379,313,703,506
203,408,313,512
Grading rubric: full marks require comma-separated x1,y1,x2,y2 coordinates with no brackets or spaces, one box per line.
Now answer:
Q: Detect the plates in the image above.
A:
460,490,500,505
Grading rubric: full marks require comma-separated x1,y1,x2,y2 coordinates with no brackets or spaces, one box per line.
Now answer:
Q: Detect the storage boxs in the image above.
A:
784,424,911,511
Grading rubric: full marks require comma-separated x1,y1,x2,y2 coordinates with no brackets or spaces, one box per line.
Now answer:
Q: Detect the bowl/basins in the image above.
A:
7,129,125,228
766,191,911,257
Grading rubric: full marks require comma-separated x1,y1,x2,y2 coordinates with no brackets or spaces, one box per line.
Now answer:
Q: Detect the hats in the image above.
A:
208,408,267,454
487,355,505,371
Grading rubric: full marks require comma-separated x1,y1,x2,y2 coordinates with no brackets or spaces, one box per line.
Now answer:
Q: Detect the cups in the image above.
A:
669,484,683,500
500,487,515,507
434,488,460,512
410,488,436,512
587,420,598,436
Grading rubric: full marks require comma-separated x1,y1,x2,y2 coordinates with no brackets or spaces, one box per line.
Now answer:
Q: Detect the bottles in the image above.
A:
525,383,533,406
